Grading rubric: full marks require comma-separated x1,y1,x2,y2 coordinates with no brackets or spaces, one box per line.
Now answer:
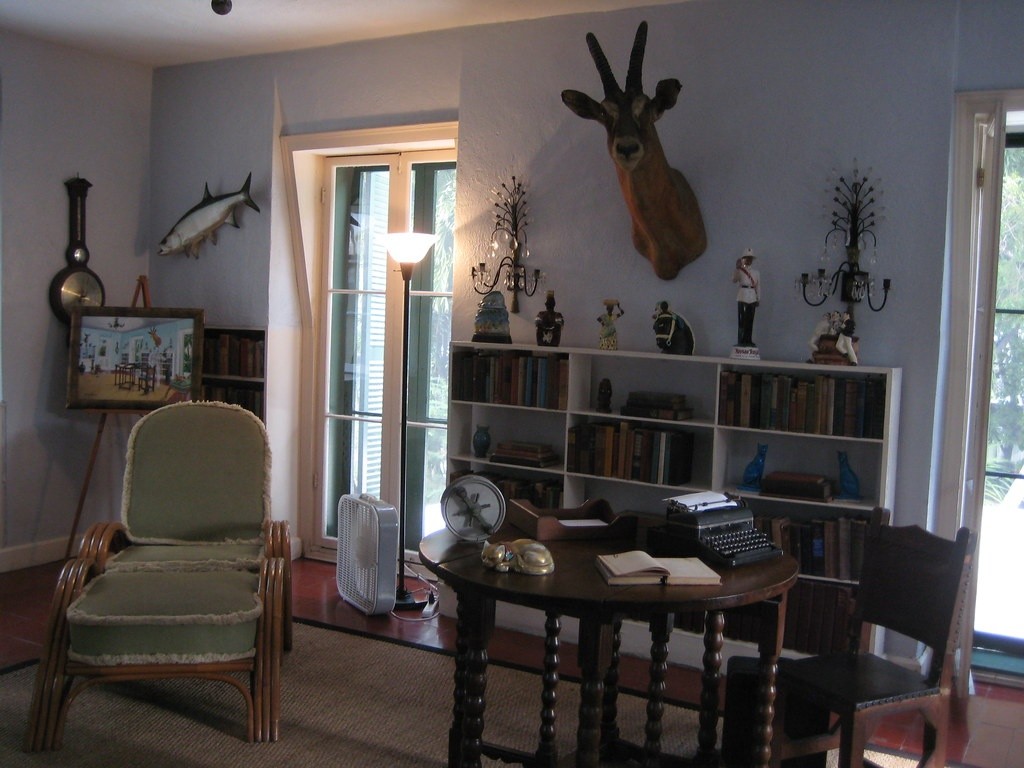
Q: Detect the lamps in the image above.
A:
379,232,439,613
786,158,892,320
467,175,547,314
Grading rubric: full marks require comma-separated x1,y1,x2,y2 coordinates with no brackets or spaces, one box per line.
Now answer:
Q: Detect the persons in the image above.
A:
597,300,624,351
734,246,761,348
808,308,859,364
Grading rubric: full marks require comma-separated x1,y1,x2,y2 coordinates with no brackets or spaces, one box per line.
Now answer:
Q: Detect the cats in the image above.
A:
481,538,555,574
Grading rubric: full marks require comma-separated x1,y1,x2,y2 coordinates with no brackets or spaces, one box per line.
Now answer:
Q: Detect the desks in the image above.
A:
419,508,803,768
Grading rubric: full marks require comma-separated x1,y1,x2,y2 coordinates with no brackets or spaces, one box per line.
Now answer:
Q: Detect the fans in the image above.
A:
334,494,399,618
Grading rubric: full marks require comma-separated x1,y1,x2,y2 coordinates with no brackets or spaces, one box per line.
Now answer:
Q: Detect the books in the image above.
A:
448,470,563,511
759,468,835,502
452,347,569,411
489,439,557,468
595,550,723,588
717,369,884,440
620,389,695,422
567,421,694,486
725,513,874,657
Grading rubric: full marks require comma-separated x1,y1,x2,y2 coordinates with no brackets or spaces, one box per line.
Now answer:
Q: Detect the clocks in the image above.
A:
50,171,105,347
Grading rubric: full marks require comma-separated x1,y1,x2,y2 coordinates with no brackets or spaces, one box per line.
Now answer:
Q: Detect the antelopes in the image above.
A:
560,18,708,280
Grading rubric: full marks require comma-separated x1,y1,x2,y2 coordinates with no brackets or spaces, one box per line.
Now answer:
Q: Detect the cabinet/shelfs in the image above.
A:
200,324,269,430
447,339,904,679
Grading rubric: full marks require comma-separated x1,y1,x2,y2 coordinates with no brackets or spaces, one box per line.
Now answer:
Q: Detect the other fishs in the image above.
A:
157,170,263,260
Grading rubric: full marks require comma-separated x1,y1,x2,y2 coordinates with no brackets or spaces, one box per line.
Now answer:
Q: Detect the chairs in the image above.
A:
718,506,978,768
21,399,293,753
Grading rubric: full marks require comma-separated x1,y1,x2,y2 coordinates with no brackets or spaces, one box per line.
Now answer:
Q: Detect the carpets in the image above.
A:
0,614,982,768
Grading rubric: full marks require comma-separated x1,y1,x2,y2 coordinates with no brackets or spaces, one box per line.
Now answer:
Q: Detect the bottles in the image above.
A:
472,423,494,458
534,290,564,347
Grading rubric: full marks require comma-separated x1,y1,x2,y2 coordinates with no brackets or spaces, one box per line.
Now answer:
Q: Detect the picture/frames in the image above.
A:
63,303,204,411
